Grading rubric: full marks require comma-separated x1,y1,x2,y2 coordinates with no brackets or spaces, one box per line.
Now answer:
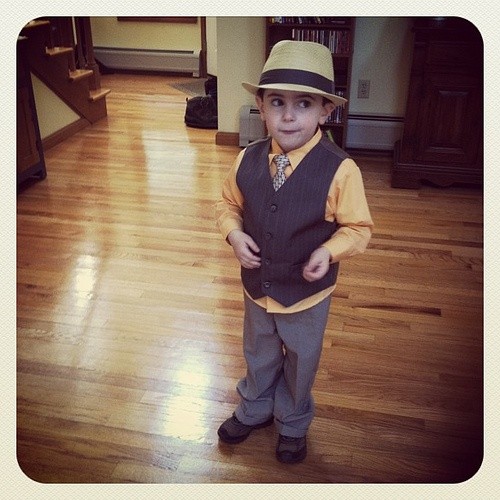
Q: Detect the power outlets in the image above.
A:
357,80,370,98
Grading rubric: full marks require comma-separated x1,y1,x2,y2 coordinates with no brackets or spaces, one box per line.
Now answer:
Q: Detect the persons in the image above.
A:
214,39,374,462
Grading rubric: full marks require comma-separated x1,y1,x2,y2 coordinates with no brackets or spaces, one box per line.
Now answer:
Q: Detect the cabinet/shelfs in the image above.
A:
264,16,355,151
390,17,484,190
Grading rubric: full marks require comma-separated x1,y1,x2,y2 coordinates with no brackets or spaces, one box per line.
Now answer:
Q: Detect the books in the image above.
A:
268,16,325,24
325,91,344,124
292,28,349,54
326,129,334,142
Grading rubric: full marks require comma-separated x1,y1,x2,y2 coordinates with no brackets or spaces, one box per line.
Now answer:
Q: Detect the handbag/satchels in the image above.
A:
184,96,218,128
204,76,217,95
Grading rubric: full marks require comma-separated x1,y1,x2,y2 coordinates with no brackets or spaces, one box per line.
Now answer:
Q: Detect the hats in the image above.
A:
241,40,348,107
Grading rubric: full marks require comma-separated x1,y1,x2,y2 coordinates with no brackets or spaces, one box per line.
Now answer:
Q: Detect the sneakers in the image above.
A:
276,433,307,464
217,416,275,444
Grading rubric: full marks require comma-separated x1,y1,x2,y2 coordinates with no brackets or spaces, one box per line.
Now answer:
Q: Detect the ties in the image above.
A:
272,154,290,192
